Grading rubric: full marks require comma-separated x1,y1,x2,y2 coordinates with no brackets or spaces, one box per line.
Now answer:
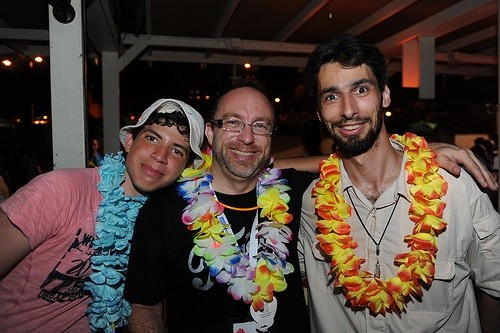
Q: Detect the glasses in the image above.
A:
211,118,275,135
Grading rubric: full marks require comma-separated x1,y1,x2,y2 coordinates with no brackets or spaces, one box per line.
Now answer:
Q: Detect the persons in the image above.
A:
88,138,103,167
296,34,500,333
0,98,206,333
123,84,500,333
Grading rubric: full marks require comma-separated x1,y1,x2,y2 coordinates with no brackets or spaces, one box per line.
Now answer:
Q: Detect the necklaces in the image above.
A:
344,186,400,278
178,148,294,312
312,132,449,315
216,197,259,212
84,151,148,332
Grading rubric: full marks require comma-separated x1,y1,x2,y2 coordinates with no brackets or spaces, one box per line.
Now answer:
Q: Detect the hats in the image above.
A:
119,98,205,170
